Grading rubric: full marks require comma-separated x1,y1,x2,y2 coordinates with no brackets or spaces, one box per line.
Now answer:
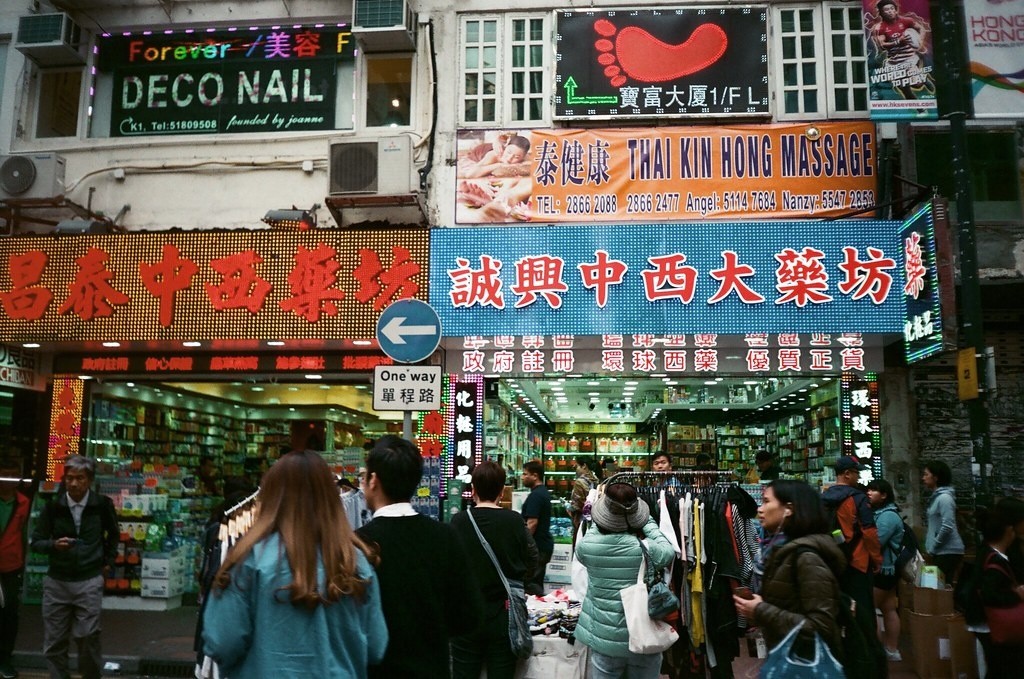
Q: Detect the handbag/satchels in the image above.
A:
638,538,680,619
977,552,1024,643
505,578,533,659
619,550,680,654
758,618,846,679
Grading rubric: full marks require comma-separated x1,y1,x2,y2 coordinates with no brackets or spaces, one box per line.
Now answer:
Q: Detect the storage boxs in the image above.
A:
897,557,980,679
142,552,190,599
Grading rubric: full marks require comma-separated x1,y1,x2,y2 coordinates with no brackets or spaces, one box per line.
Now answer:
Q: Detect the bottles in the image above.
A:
150,497,224,591
96,475,183,498
832,529,845,544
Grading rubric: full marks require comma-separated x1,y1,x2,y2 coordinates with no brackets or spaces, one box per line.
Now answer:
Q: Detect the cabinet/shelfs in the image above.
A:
482,376,844,488
80,394,363,496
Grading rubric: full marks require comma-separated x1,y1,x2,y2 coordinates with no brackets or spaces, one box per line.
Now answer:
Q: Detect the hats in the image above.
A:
591,482,651,534
835,455,867,476
756,450,779,465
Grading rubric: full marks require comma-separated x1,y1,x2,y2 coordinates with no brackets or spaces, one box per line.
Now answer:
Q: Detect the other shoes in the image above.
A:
884,648,902,661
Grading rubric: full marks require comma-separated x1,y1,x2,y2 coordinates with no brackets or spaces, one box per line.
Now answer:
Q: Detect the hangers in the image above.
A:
613,468,735,508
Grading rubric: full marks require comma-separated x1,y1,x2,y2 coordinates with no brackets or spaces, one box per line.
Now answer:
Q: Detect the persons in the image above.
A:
450,461,538,679
520,462,553,598
572,482,675,679
32,455,120,679
820,455,883,678
652,450,682,495
864,479,904,661
201,449,390,679
966,496,1024,679
755,451,779,480
351,435,478,679
566,455,615,562
0,459,31,679
733,480,843,660
193,459,264,679
874,1,935,113
196,453,216,495
456,133,532,223
693,454,718,493
923,460,965,585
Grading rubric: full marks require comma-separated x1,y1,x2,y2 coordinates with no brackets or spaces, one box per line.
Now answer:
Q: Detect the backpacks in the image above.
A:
880,511,921,583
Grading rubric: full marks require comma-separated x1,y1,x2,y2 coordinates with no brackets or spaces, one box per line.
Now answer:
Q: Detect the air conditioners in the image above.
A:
351,0,415,54
328,133,420,204
0,152,68,202
14,12,88,67
